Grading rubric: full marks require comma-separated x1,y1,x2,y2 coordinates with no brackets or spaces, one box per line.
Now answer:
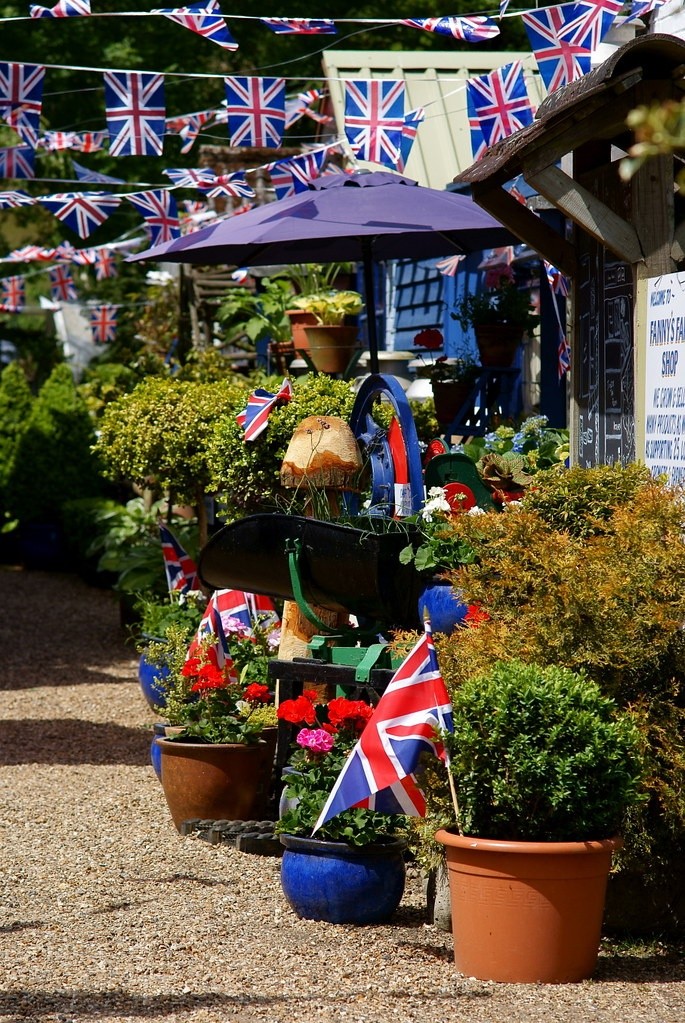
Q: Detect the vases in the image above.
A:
150,735,270,839
430,381,476,424
137,653,199,716
150,722,172,787
278,827,408,928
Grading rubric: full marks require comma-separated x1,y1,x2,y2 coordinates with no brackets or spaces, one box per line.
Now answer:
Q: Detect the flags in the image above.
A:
312,620,454,837
235,377,292,441
182,589,282,692
159,517,200,594
0,0,664,384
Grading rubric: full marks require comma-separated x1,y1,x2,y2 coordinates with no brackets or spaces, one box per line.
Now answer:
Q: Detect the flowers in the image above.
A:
137,585,415,840
451,263,542,336
415,328,475,382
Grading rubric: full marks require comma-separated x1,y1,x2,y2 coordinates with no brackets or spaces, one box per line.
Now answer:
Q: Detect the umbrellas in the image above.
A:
125,169,522,406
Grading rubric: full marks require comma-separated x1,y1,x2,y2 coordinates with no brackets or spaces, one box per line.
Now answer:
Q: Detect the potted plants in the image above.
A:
277,262,354,356
413,655,653,984
288,285,366,374
477,329,520,367
